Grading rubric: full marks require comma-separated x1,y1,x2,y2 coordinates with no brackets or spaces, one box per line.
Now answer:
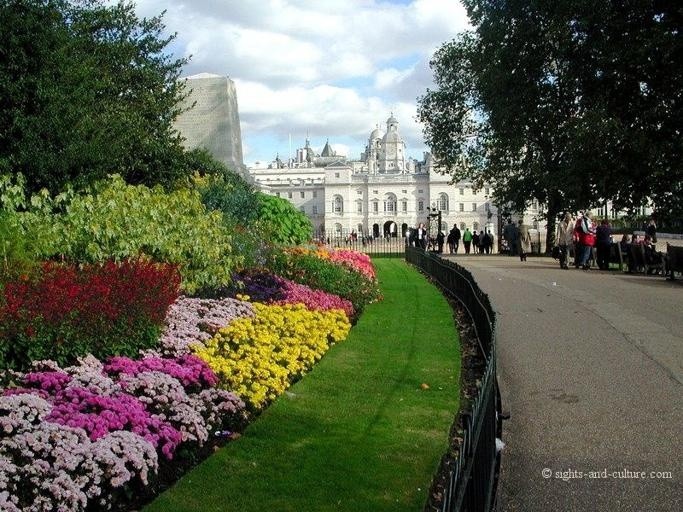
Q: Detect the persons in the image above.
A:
345,230,373,246
408,211,668,275
385,231,390,242
322,236,326,245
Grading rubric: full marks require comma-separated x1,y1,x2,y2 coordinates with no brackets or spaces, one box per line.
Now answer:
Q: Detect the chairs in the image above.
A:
573,242,683,283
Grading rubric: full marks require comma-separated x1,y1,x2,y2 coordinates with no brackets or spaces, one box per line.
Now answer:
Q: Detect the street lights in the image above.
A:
429,207,442,253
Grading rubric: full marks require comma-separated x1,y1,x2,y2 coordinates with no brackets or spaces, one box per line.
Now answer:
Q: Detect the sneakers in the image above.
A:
560,263,591,270
